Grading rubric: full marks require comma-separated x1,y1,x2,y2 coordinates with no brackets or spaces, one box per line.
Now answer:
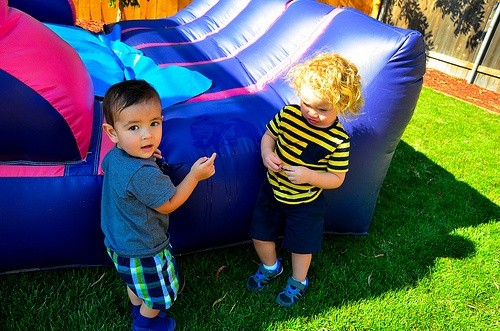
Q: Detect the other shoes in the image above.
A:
131,314,176,331
131,304,166,319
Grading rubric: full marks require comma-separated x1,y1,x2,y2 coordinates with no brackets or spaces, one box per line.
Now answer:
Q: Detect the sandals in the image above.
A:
276,276,309,307
247,258,284,292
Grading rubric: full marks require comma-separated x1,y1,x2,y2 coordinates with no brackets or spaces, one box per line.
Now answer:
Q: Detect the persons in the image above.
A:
246,55,362,306
100,80,218,331
